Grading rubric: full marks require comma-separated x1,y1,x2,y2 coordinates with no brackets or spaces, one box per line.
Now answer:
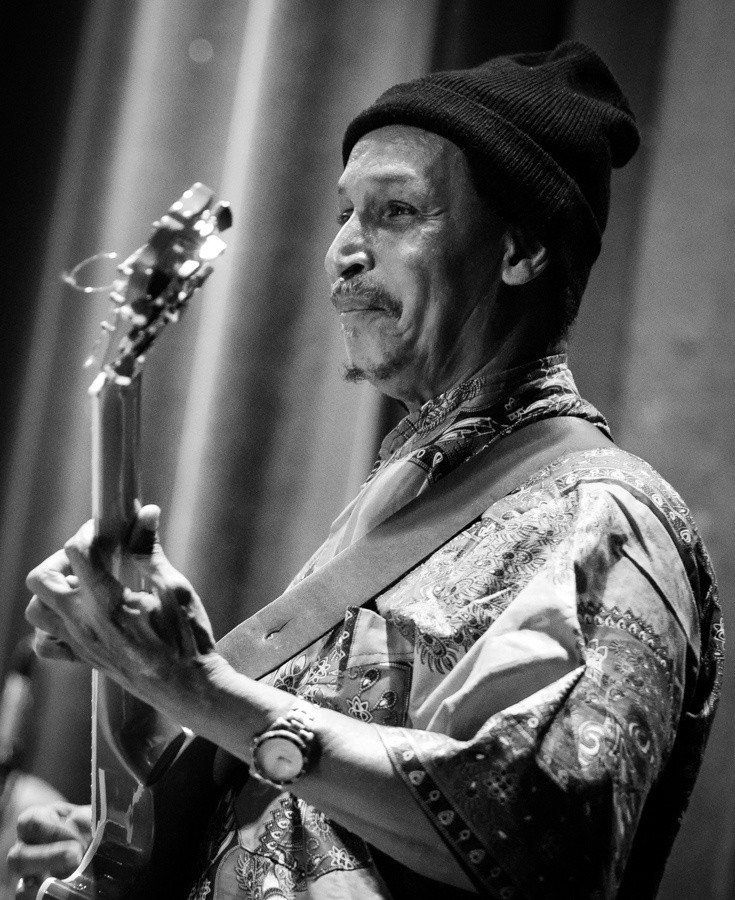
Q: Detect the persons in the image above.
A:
1,41,726,900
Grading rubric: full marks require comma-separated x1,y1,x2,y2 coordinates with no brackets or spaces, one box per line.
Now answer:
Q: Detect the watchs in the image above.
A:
246,694,327,789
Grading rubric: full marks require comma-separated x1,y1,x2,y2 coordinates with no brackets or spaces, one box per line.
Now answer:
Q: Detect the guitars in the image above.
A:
0,182,240,900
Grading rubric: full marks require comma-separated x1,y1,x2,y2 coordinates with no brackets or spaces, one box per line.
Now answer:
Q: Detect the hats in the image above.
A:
342,40,641,267
